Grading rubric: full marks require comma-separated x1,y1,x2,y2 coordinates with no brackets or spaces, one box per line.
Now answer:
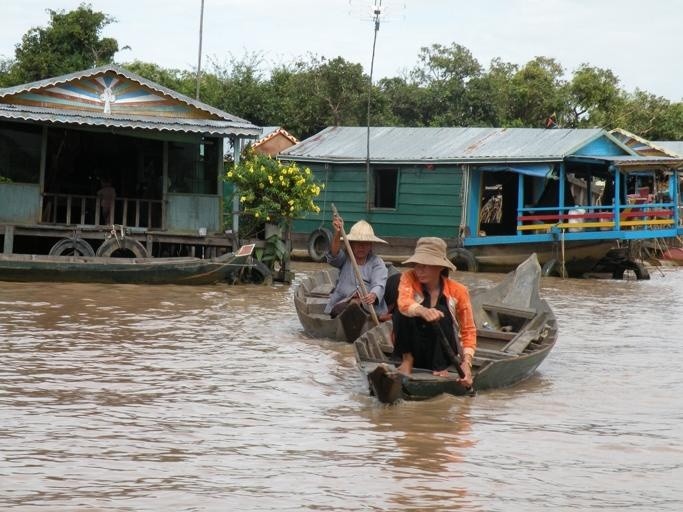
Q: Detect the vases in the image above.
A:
263,208,282,240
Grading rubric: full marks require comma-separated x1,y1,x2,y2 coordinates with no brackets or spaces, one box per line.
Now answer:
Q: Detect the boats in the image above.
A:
0,244,256,285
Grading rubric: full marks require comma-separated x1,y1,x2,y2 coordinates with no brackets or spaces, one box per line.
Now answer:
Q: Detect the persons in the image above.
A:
389,234,477,389
324,214,390,321
95,180,116,225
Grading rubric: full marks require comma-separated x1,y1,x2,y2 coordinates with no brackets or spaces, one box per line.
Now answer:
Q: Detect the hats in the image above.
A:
400,237,457,273
338,218,388,250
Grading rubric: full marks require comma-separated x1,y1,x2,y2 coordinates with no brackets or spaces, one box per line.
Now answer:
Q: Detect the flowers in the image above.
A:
215,141,325,228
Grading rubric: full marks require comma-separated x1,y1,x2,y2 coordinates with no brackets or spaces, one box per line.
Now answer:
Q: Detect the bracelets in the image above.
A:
463,358,472,367
